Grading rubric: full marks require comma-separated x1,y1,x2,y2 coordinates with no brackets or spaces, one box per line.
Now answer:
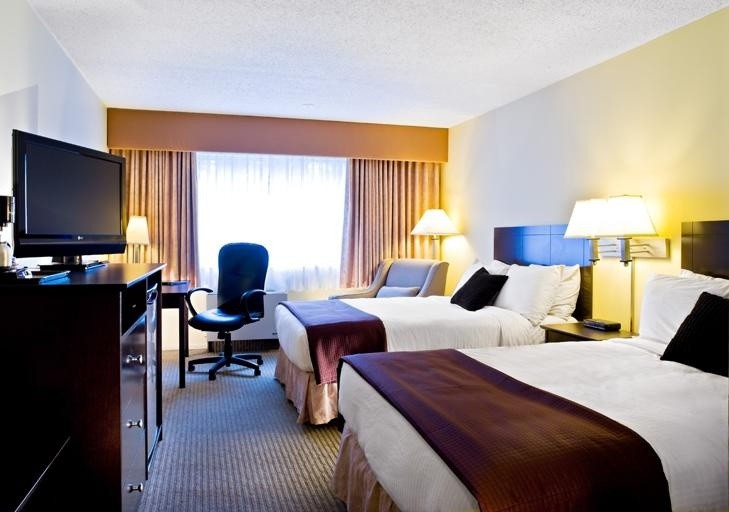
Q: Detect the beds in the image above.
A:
273,225,593,426
332,219,729,512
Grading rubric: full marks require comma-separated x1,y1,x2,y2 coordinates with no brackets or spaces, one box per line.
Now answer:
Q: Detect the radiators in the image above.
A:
205,291,287,353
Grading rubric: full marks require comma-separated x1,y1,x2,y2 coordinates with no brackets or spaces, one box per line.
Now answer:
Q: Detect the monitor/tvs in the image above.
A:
12,129,127,273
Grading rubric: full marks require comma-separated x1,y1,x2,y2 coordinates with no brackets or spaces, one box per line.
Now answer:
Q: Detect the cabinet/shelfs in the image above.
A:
0,262,168,512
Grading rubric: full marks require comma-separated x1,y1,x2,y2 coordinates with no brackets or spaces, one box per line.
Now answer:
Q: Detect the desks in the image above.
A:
162,280,191,388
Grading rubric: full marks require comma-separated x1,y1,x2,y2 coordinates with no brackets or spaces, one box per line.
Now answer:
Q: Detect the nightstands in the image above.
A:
540,323,639,344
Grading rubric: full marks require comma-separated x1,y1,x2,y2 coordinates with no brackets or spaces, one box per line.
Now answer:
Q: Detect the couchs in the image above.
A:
327,258,449,298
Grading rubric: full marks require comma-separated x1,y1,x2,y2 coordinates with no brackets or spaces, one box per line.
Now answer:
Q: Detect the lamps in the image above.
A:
125,216,149,245
411,207,456,260
563,195,671,265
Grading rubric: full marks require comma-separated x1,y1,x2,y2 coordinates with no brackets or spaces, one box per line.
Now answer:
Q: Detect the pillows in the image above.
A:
494,264,561,327
452,266,508,311
677,267,729,300
530,264,582,326
638,272,724,347
661,290,728,378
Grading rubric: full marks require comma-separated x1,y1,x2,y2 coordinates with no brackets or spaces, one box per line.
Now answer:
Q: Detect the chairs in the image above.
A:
186,242,269,380
